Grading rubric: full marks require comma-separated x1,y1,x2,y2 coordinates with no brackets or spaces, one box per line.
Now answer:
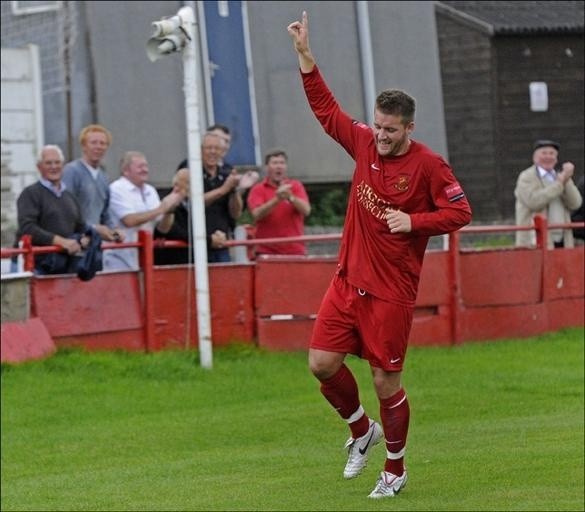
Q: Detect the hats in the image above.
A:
532,140,559,152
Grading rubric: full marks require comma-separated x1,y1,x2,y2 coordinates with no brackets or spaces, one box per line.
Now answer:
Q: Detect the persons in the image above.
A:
570,176,585,245
15,142,92,276
62,124,128,274
244,146,312,258
208,124,260,262
200,132,243,264
154,166,195,267
285,8,472,502
100,147,187,273
512,139,584,252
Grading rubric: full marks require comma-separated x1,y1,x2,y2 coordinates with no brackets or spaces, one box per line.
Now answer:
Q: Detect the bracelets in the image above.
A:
231,187,240,196
165,207,177,215
286,196,295,205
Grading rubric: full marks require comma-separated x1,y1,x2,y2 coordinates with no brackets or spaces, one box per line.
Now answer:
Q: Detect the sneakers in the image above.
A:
344,418,384,480
368,464,407,499
